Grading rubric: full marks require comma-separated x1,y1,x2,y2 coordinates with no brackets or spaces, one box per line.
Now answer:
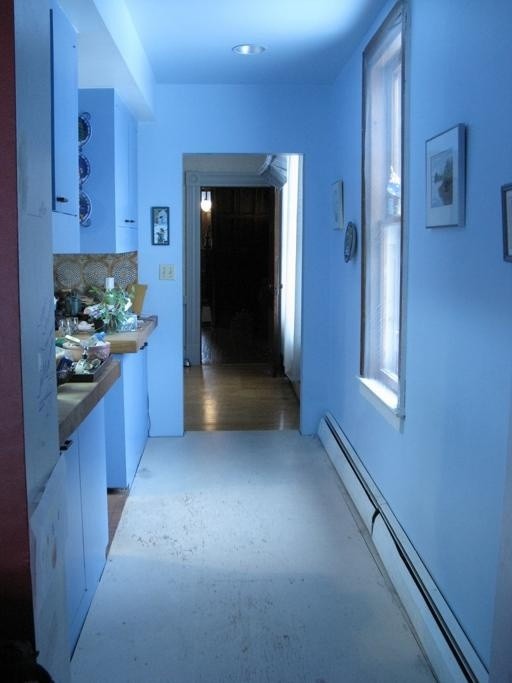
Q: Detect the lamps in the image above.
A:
200,190,213,214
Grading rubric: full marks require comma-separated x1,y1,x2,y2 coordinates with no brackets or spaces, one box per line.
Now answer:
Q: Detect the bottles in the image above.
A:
102,276,118,330
71,297,80,316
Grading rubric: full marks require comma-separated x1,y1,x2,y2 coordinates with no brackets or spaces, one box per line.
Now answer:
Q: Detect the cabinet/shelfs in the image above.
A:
0,1,152,662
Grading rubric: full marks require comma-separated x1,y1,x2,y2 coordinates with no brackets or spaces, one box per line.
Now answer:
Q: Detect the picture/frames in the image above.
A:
424,121,465,228
497,182,512,263
150,206,171,247
330,181,344,232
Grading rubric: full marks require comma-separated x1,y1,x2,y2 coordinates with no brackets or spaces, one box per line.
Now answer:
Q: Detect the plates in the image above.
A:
79,153,91,185
80,191,92,227
78,113,93,149
342,220,357,264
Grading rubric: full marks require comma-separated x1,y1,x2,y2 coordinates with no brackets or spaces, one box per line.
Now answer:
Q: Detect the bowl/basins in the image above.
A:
86,342,112,363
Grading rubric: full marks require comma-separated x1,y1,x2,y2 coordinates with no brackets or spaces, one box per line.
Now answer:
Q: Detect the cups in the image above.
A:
57,316,79,338
